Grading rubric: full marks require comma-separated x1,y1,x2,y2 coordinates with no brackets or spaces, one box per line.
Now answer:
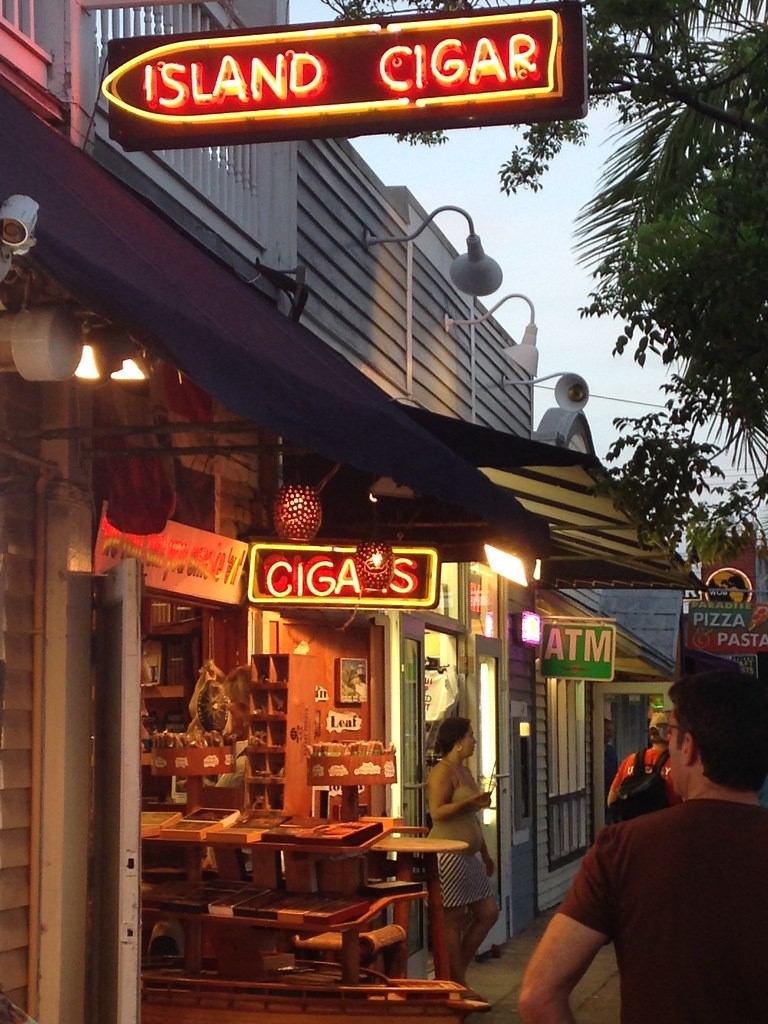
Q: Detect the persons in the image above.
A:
426,716,500,1003
605,710,686,824
516,668,768,1024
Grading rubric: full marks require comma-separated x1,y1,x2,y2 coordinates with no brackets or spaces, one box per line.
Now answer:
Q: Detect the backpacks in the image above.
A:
616,746,670,821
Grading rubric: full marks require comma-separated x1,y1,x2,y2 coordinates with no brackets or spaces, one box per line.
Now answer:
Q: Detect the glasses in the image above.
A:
656,722,678,738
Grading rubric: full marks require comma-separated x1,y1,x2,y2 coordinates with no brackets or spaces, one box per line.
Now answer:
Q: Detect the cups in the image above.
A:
478,778,497,802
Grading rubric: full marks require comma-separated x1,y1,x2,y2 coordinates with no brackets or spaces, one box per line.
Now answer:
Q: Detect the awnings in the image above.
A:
400,395,710,593
1,88,515,513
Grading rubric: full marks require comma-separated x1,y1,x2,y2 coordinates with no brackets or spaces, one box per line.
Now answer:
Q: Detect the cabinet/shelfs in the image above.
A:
139,590,467,989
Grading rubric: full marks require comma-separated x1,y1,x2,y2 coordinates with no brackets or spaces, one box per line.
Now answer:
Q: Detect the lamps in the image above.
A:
483,533,535,588
445,293,539,377
502,372,589,411
356,535,393,592
272,482,322,538
364,206,503,296
